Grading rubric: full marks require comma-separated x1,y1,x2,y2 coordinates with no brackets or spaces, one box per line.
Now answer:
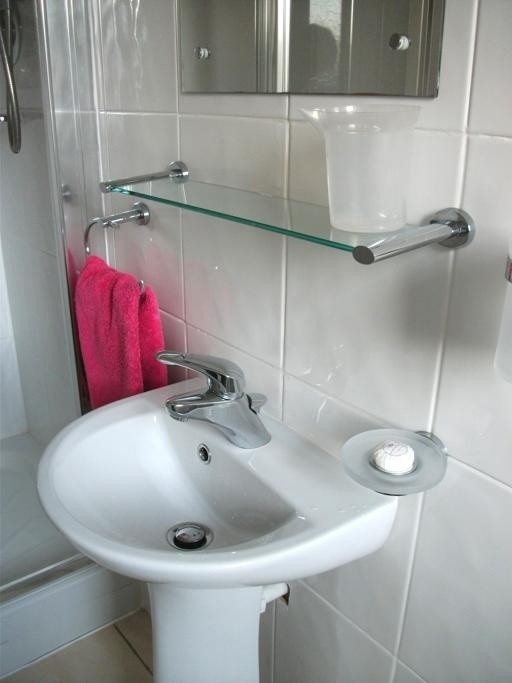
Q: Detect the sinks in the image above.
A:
35,376,399,587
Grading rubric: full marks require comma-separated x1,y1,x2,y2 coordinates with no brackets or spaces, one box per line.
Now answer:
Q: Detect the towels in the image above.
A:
71,254,170,410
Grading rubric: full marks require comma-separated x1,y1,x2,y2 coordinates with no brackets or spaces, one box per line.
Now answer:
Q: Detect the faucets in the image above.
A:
154,350,272,448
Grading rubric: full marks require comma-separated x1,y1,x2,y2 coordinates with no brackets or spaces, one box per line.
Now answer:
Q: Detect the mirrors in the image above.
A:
176,0,445,99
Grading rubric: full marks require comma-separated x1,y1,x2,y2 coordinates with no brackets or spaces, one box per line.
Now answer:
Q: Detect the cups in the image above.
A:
299,102,423,236
